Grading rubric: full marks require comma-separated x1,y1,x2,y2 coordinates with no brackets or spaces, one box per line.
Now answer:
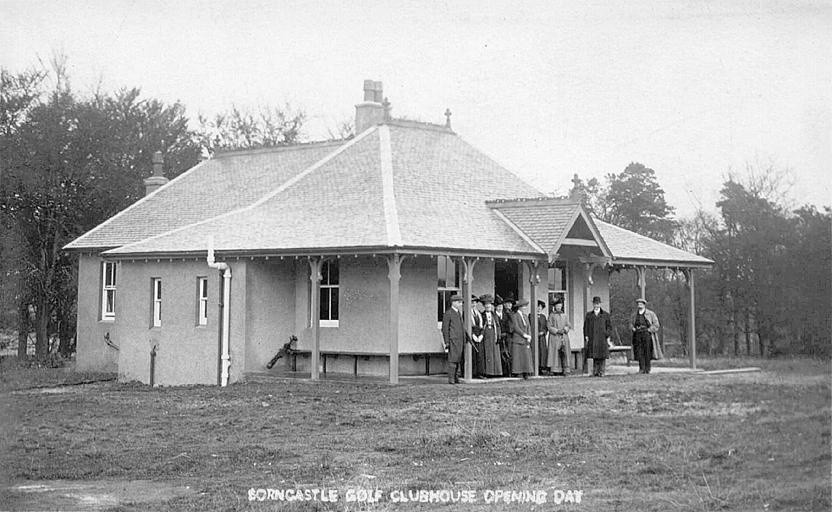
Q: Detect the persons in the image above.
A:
583,296,612,377
441,294,468,385
528,300,549,375
629,298,663,374
546,298,573,376
463,294,535,380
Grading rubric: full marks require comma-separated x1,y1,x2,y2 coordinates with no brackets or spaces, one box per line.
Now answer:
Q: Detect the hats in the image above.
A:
635,299,649,305
591,297,603,304
450,294,565,309
